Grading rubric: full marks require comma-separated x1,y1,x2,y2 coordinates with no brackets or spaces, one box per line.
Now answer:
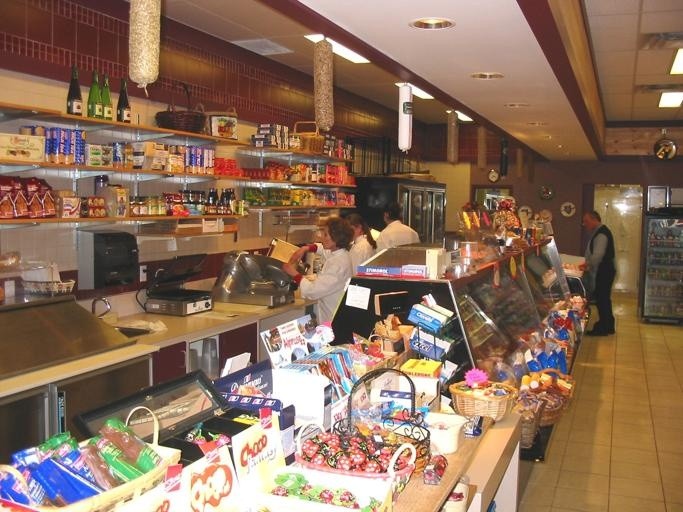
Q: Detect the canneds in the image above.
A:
139,201,148,216
159,194,168,215
149,195,159,214
130,202,139,217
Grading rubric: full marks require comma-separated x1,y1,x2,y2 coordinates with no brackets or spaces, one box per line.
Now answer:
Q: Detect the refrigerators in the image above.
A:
638,212,682,325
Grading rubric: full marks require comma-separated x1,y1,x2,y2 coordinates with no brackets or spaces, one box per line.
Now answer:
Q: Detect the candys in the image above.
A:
303,484,311,493
273,483,288,497
320,489,335,503
339,491,353,504
305,432,410,474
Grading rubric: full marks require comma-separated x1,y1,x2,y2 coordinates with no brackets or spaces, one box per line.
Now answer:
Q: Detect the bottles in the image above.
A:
647,285,683,298
87,70,103,119
649,251,682,266
101,74,113,120
651,230,683,247
117,77,131,123
178,188,206,215
647,302,683,316
208,187,235,214
67,66,83,116
649,268,682,281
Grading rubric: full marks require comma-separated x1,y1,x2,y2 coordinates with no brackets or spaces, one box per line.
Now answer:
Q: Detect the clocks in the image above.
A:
487,169,499,182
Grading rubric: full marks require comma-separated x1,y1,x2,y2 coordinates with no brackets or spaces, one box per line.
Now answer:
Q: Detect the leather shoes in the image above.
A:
609,328,614,334
586,329,609,337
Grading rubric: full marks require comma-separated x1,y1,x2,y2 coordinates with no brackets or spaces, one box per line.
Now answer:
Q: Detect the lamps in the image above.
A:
666,46,683,75
470,72,504,80
409,17,457,31
656,88,683,110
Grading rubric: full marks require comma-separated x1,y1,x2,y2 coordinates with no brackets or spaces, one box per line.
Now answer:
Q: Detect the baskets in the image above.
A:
536,368,575,410
528,387,566,426
291,120,326,156
1,405,180,511
196,104,238,141
296,422,416,502
511,395,547,448
156,82,206,133
333,368,431,474
450,381,517,421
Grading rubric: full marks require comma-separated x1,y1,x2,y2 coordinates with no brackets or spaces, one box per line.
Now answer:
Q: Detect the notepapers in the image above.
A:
345,284,371,310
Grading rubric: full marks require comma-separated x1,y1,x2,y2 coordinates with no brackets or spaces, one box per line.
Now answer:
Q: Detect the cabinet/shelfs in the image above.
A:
252,179,357,187
238,146,355,163
1,158,251,180
28,112,253,147
249,205,356,209
0,214,241,224
1,345,164,469
118,296,316,388
330,235,590,462
356,177,446,244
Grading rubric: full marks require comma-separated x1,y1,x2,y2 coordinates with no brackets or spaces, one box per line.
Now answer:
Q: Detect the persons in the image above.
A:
375,201,420,254
282,217,353,327
345,214,377,275
579,210,616,336
